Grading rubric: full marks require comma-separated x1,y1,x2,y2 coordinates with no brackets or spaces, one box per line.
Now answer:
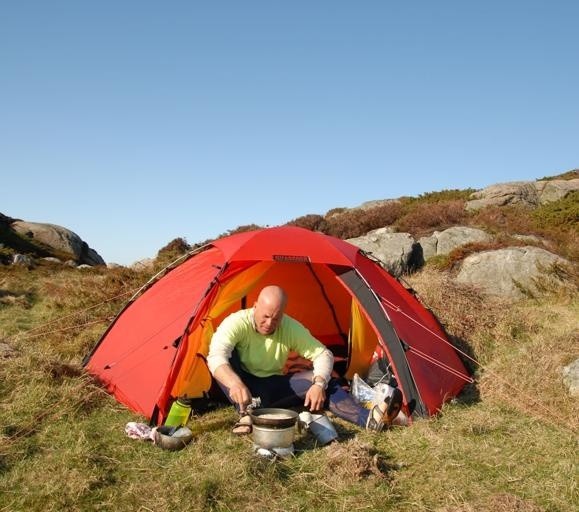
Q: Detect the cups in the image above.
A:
309,415,340,444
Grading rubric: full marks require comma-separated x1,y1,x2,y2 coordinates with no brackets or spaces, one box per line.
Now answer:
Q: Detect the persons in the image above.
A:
205,284,403,436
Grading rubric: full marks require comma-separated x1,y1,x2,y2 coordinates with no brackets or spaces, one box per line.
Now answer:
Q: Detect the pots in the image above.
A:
236,407,299,428
249,422,296,449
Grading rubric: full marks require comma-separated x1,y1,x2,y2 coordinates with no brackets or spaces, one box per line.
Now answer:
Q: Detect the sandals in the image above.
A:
369,388,403,435
232,414,253,436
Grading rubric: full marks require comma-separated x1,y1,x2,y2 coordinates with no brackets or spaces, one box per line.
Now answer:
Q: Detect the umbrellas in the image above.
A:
80,223,472,429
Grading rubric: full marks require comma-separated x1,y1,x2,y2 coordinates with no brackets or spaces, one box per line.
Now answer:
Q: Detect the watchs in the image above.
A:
314,380,329,390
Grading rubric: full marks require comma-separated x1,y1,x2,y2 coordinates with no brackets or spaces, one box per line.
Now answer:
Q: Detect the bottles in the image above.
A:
162,395,193,430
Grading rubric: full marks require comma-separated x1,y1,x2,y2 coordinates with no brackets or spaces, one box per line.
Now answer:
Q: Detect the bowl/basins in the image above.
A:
153,426,194,451
